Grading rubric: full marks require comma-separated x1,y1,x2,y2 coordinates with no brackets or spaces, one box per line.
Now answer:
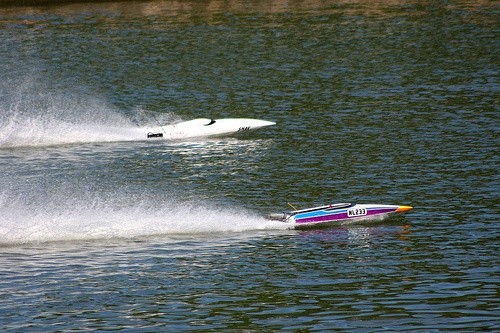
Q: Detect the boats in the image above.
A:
126,118,278,142
266,203,415,230
